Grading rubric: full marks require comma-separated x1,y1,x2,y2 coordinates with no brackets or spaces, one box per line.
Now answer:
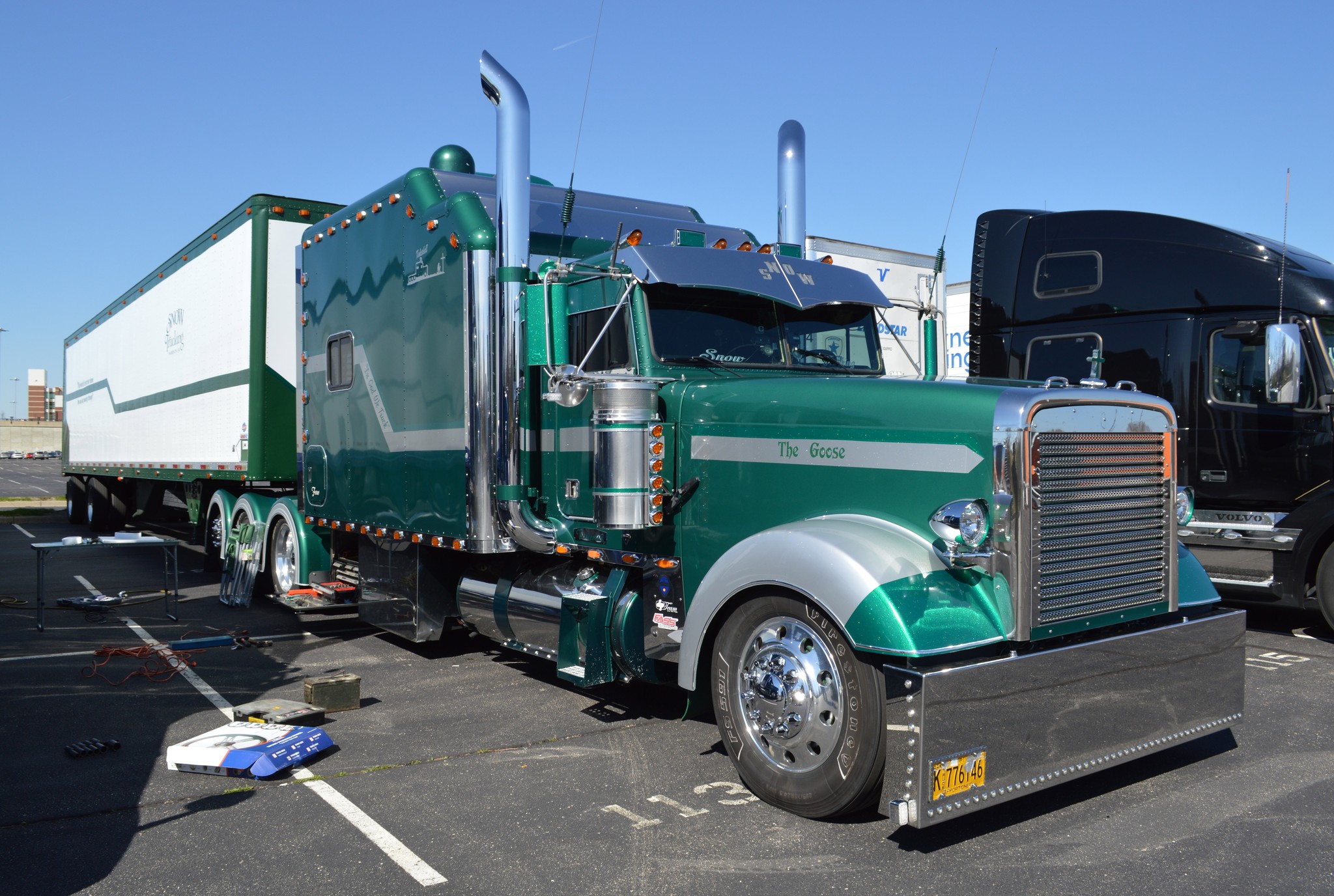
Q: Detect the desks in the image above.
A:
31,538,180,633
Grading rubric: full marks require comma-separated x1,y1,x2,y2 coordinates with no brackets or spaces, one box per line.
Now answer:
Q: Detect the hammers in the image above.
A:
248,639,273,647
235,636,251,647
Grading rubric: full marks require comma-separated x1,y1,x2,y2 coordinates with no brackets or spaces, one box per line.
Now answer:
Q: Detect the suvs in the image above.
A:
32,451,44,460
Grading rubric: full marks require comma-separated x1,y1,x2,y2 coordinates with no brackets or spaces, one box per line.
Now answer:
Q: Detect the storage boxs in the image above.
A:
303,672,362,713
166,722,335,781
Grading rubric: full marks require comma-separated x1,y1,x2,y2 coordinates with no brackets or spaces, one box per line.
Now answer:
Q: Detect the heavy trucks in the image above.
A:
771,206,1333,626
57,52,1250,830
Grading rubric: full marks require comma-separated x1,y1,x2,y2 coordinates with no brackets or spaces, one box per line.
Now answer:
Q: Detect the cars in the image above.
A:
26,452,33,459
42,450,62,460
6,451,15,458
23,452,29,459
0,452,10,460
11,452,23,459
